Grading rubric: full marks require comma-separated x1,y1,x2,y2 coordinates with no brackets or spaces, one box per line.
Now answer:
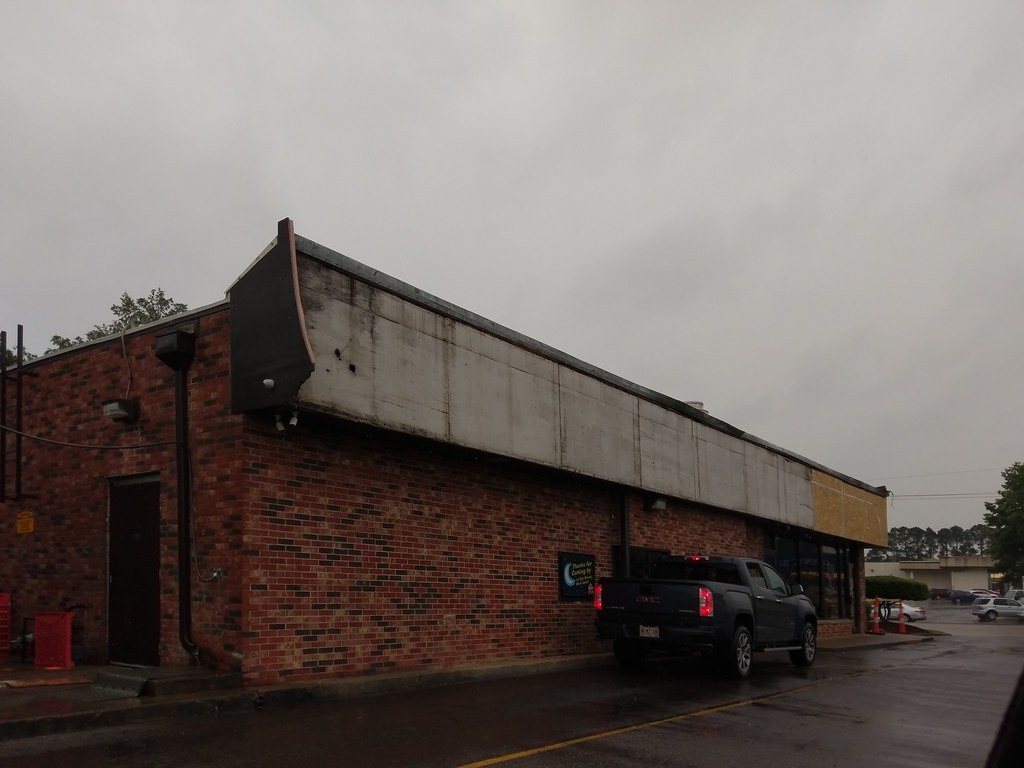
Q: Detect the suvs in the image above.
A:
971,597,1024,621
1004,589,1024,605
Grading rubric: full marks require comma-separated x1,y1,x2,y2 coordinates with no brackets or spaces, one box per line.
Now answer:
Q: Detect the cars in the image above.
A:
867,599,927,623
930,588,1002,605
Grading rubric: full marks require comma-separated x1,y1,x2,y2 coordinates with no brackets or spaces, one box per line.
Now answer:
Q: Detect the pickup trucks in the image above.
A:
592,554,818,681
